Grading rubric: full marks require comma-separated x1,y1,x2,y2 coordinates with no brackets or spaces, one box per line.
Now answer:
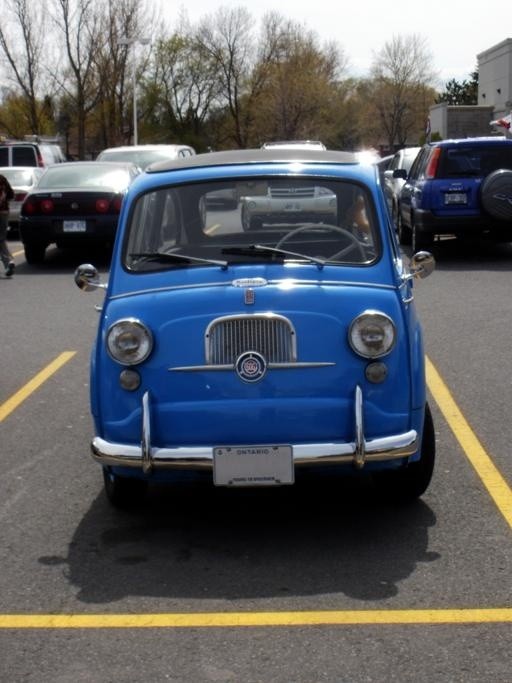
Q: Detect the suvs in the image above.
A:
395,137,511,254
240,137,344,232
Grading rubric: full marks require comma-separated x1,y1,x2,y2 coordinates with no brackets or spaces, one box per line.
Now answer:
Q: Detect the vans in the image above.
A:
0,140,69,171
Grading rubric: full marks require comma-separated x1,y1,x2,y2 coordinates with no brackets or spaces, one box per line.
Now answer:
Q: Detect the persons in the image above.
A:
0,174,16,277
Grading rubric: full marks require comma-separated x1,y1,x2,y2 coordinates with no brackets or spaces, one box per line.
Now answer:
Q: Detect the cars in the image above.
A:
73,149,441,505
22,159,141,262
0,165,51,240
93,143,209,238
383,146,421,231
